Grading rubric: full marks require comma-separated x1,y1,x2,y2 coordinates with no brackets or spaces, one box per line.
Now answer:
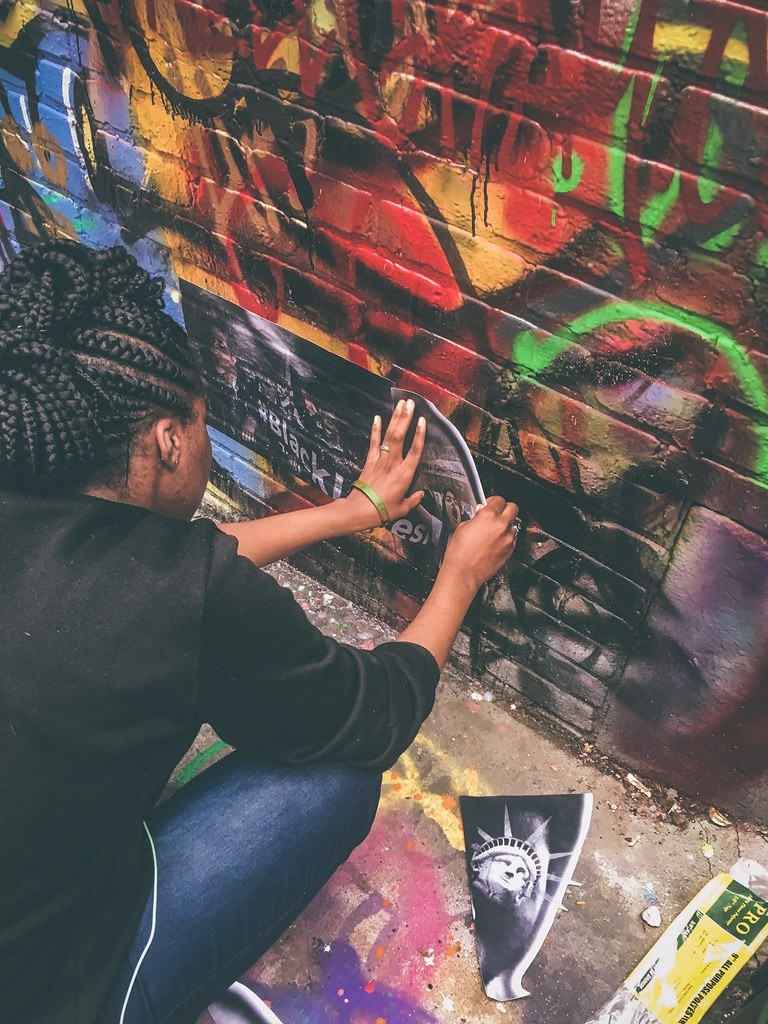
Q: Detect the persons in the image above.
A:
469,837,541,909
415,490,462,547
0,237,523,1024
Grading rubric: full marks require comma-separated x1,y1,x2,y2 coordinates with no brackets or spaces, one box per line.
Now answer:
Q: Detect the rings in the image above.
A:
512,521,521,537
380,446,389,451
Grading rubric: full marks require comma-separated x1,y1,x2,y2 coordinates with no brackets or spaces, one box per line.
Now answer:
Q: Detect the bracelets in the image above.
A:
351,480,391,527
